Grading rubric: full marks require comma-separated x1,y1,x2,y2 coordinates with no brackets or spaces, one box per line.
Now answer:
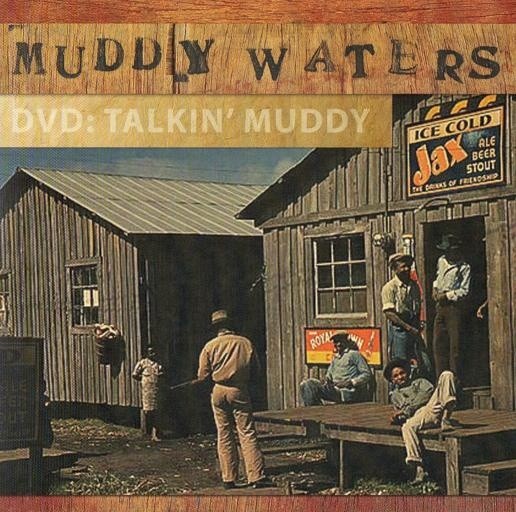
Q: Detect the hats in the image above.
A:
330,333,349,342
384,360,411,382
211,309,228,325
388,253,412,270
436,235,464,250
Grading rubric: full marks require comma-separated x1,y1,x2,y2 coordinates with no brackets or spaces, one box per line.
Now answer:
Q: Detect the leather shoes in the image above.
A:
224,483,234,490
252,483,263,488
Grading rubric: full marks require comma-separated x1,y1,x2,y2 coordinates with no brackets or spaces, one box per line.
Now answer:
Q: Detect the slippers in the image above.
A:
409,471,428,486
441,419,455,431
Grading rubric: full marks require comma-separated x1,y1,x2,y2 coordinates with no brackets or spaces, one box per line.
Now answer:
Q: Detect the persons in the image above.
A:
476,299,488,322
196,309,278,490
430,233,472,389
299,330,372,437
131,344,164,443
381,252,436,391
382,358,464,486
